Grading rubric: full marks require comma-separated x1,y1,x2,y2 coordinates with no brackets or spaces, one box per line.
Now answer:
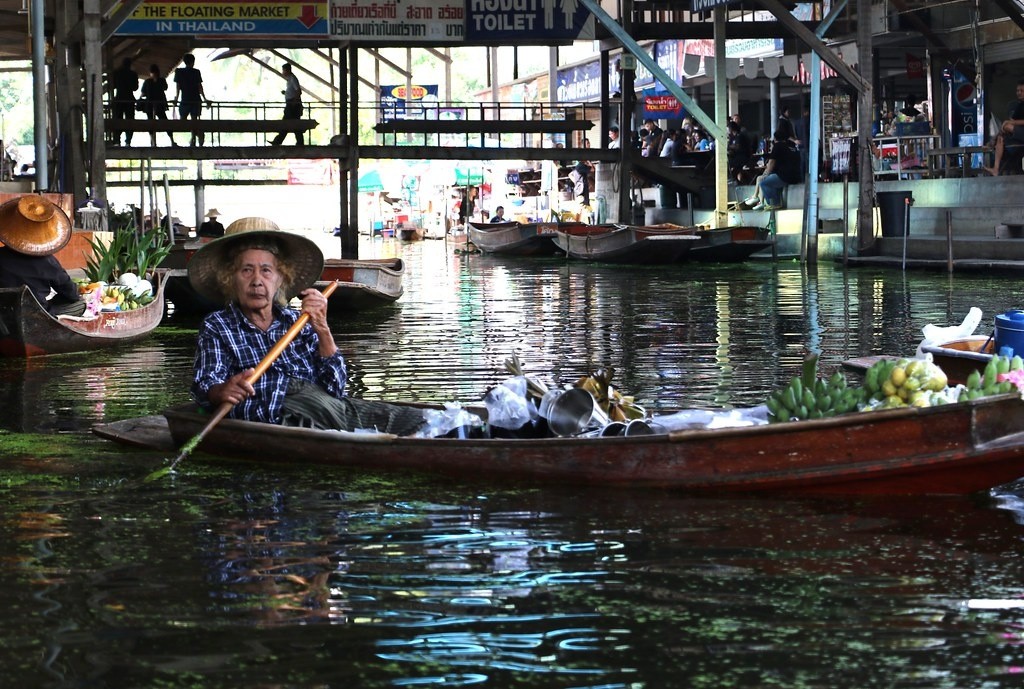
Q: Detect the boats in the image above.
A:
692,227,773,257
465,221,548,259
549,225,703,262
159,392,1023,506
171,257,405,307
0,272,172,351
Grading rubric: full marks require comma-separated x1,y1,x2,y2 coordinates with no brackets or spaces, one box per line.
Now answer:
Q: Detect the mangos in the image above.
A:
79,283,102,293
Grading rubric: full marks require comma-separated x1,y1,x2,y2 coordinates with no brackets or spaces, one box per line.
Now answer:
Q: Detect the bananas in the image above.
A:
930,353,1023,405
101,285,155,310
766,353,899,424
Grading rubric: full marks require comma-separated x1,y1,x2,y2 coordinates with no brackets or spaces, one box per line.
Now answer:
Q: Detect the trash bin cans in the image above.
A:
876,191,912,237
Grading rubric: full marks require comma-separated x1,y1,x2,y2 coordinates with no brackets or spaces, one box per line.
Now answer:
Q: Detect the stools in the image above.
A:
998,143,1024,175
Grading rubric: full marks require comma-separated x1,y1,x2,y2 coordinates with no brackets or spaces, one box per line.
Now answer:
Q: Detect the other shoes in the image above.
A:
764,205,782,209
753,204,766,210
745,197,760,205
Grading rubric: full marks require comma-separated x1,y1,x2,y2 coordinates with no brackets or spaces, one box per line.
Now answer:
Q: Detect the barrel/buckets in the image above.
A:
876,191,914,238
599,420,668,436
993,310,1024,359
546,388,610,437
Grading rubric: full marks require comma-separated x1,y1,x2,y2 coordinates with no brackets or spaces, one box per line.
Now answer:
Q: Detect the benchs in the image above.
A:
929,146,993,178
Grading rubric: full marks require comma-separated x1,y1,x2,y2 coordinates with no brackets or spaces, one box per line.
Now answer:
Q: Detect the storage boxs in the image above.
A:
882,143,906,158
895,121,931,135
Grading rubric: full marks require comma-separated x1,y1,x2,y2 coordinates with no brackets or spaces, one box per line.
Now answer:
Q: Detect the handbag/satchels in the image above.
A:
136,93,152,113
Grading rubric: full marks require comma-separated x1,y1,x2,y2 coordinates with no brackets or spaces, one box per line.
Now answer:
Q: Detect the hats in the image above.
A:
0,194,72,255
205,209,221,217
189,217,324,308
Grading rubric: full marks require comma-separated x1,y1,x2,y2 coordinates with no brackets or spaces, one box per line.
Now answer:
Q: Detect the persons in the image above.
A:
490,206,506,223
726,114,749,185
141,64,178,147
0,139,17,182
266,63,304,145
631,118,716,165
199,209,225,239
109,58,140,146
174,54,213,147
187,217,425,435
555,138,594,208
885,95,921,136
459,186,476,225
608,126,620,192
983,83,1024,176
759,103,810,211
745,141,775,210
120,204,190,237
0,195,87,319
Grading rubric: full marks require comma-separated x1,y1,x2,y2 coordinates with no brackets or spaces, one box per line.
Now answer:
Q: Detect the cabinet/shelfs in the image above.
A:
871,135,943,180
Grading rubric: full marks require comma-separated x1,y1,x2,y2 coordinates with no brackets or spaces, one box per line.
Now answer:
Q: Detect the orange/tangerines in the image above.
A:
860,359,947,413
